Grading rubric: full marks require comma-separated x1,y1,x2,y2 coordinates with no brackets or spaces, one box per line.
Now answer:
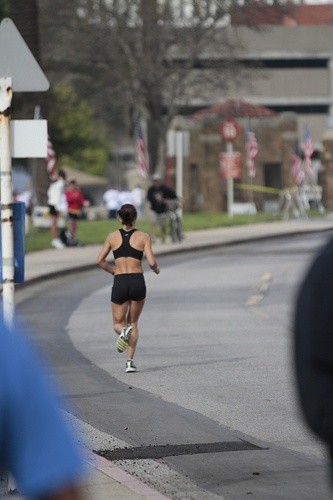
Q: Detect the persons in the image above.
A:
148,175,182,244
64,179,90,247
46,169,67,249
12,165,36,236
0,298,95,500
96,204,160,372
103,183,145,220
291,153,333,489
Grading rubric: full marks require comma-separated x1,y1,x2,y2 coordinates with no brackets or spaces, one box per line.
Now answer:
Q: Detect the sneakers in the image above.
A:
124,359,136,373
116,325,133,353
